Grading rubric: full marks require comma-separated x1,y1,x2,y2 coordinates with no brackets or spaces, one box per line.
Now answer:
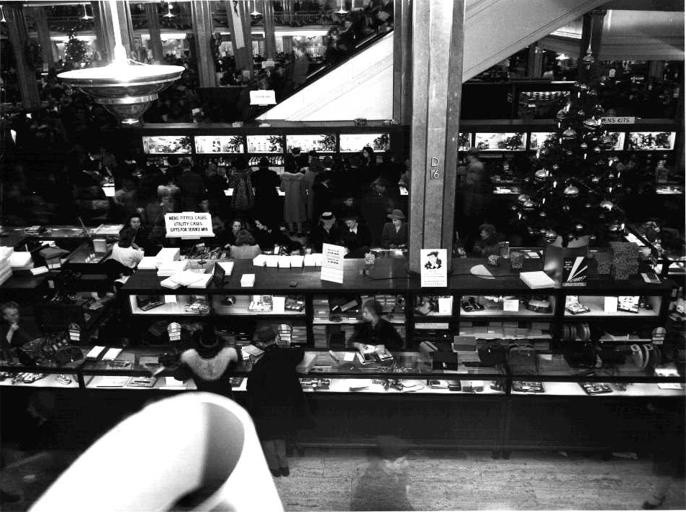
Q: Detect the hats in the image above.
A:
193,325,224,359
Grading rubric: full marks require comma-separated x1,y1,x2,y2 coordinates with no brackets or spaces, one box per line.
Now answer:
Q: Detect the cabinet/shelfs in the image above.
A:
0,114,685,457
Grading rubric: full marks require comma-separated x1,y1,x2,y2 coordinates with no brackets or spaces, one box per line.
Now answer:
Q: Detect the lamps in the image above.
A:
335,0,347,16
55,1,183,129
162,1,176,18
249,0,261,18
79,3,92,21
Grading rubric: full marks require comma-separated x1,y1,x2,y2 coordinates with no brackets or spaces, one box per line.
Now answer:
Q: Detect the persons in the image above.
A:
1,2,683,403
639,410,686,508
348,432,421,510
244,326,307,479
